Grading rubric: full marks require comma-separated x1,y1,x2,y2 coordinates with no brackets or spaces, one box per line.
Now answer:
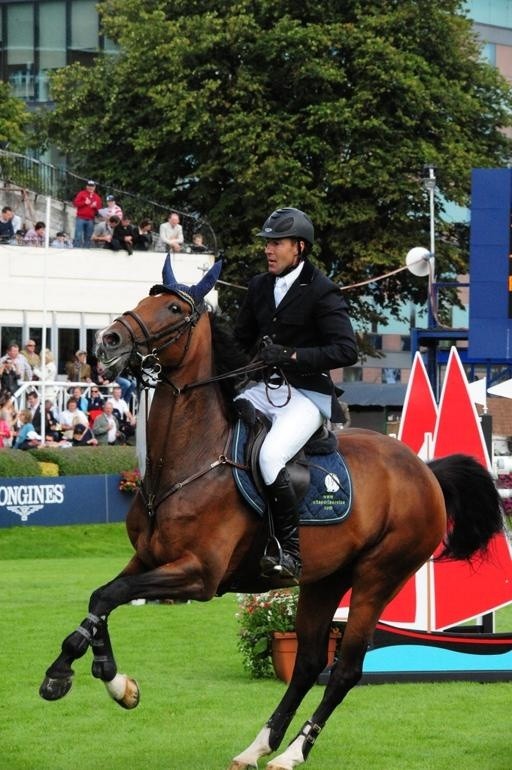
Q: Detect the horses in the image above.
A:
41,248,510,770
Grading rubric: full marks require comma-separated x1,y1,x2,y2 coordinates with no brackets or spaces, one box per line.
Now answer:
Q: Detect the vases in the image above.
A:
268,629,343,681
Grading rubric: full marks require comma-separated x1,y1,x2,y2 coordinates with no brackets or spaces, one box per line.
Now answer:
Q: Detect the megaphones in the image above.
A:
405,245,436,277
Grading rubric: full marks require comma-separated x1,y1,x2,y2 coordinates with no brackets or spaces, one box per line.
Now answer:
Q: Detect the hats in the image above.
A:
86,179,115,201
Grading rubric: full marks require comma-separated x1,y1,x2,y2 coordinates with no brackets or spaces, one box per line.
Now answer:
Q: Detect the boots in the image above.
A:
259,466,303,586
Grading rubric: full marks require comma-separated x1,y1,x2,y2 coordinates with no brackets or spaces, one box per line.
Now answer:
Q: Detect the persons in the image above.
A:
1,180,210,257
2,331,150,451
230,204,361,592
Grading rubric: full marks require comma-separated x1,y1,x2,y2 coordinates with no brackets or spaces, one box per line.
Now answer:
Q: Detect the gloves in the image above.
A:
260,344,296,369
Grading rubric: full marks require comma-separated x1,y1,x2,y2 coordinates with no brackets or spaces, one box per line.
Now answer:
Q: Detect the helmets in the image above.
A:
255,206,315,247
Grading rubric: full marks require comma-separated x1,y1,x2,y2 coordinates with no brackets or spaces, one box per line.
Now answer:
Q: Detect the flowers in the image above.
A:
235,585,339,680
118,467,143,491
